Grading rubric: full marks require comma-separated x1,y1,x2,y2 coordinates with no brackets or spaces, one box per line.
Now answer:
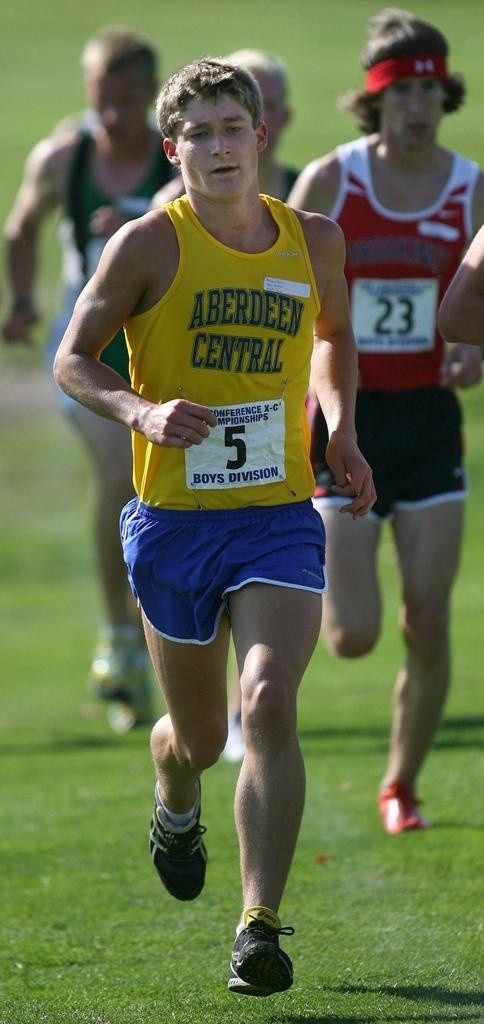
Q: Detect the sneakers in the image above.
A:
148,776,208,901
375,780,426,833
225,914,295,998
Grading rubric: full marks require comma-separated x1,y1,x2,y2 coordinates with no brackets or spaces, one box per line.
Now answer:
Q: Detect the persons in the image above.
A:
285,2,484,834
437,222,484,343
147,48,305,765
54,49,378,995
0,26,179,729
